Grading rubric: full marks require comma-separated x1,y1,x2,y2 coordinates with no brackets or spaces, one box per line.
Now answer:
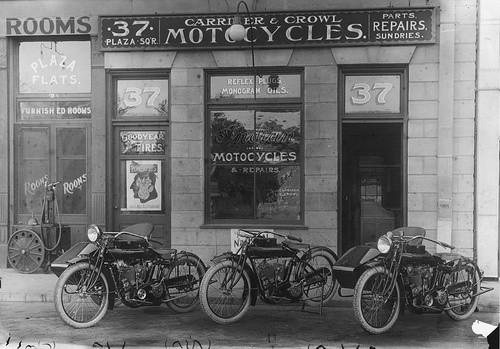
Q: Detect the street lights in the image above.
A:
228,0,260,224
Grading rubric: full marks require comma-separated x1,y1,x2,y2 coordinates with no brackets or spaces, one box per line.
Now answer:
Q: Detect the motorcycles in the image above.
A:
332,226,495,336
198,225,338,326
50,222,211,329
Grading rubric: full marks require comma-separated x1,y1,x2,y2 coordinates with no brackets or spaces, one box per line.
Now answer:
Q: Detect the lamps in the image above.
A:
229,1,256,69
